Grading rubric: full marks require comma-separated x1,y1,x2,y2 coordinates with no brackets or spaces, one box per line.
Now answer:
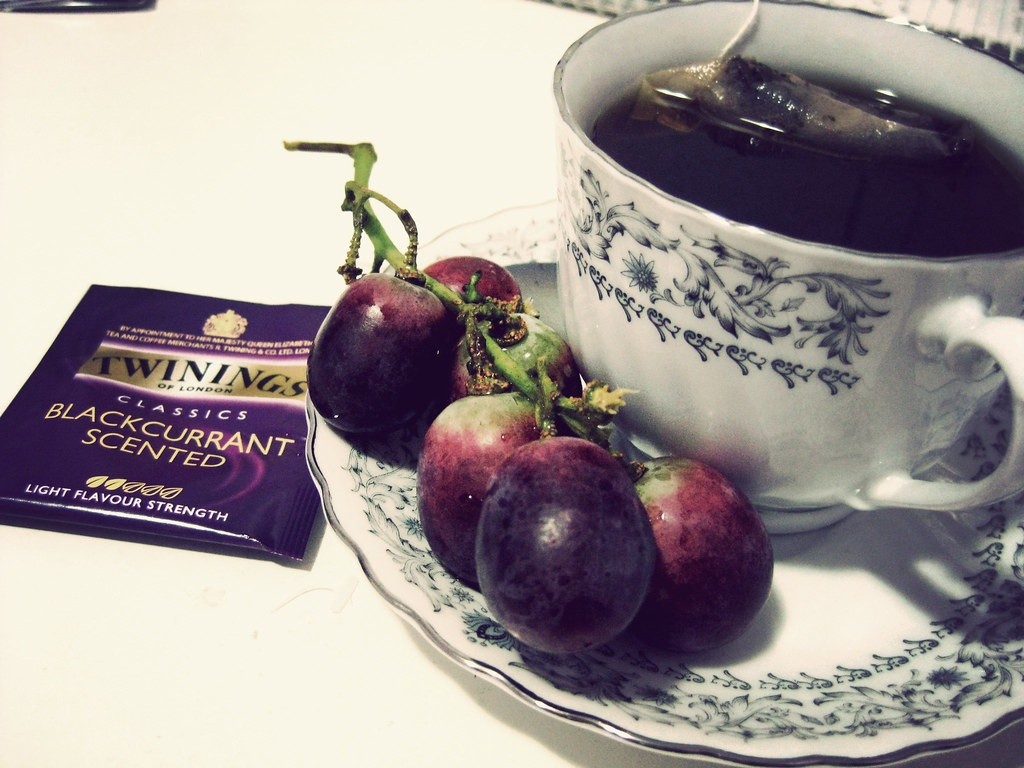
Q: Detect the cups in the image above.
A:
554,0,1024,536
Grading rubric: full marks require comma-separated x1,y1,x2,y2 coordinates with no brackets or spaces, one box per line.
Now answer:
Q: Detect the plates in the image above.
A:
305,196,1024,768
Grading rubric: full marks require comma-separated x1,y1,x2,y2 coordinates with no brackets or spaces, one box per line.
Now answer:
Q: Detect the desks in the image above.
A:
2,1,1024,768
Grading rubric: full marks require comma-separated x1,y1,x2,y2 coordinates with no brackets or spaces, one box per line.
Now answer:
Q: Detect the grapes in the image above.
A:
304,257,774,656
